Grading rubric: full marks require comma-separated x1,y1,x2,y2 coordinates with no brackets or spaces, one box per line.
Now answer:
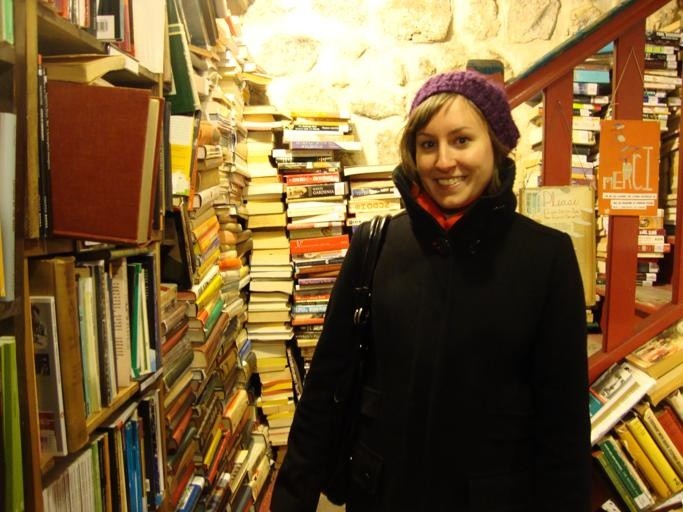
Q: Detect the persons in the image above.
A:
268,68,593,510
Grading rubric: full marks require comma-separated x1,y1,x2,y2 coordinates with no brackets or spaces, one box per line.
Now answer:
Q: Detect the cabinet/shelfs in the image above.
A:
485,4,683,512
0,3,272,512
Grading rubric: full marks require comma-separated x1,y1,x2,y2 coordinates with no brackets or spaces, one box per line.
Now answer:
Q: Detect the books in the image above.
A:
277,104,409,448
505,27,683,512
2,0,275,512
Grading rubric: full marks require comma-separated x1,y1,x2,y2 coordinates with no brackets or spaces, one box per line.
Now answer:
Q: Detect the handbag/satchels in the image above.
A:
320,215,391,507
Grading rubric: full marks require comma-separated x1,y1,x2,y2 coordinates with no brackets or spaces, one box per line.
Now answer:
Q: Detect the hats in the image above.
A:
410,71,519,152
